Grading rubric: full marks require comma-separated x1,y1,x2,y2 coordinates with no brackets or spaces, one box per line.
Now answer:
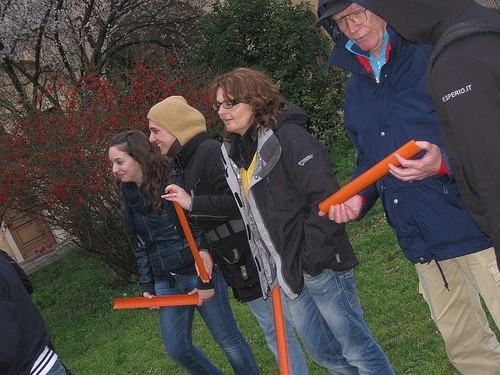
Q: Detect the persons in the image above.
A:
0,249,67,375
357,0,500,260
209,65,395,375
145,95,312,375
315,0,500,374
108,130,260,375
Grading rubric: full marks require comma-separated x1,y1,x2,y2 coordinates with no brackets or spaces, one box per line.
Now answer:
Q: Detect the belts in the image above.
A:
204,218,245,244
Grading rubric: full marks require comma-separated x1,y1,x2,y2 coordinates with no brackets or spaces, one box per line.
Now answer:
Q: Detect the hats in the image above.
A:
315,0,353,28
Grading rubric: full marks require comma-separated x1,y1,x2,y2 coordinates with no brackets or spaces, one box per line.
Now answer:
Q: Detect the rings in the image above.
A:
174,193,177,197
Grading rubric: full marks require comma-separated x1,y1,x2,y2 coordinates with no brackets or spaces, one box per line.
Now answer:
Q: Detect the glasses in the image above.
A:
212,99,240,111
330,8,366,33
